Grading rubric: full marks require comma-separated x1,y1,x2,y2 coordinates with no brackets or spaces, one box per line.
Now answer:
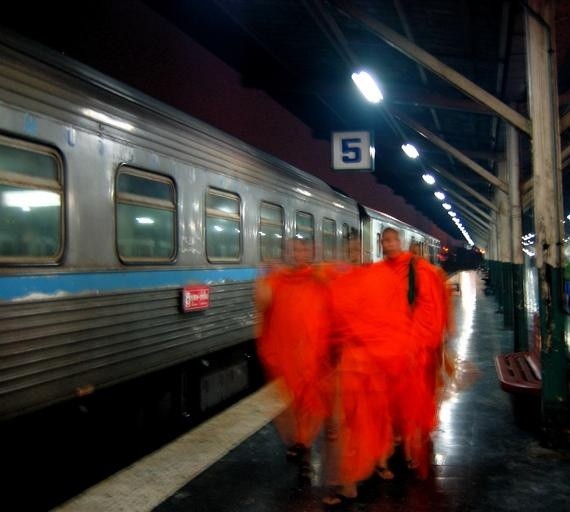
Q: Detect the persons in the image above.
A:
313,223,403,509
376,227,459,484
252,235,334,462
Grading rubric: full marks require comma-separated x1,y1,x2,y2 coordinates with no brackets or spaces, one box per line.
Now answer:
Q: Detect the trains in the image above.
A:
0,27,440,418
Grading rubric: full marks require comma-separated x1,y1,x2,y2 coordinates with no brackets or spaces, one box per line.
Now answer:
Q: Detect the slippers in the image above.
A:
322,489,359,507
374,463,395,480
284,444,301,459
298,464,317,483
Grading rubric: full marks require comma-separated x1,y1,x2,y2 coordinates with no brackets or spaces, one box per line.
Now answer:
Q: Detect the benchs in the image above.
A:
494,313,542,428
